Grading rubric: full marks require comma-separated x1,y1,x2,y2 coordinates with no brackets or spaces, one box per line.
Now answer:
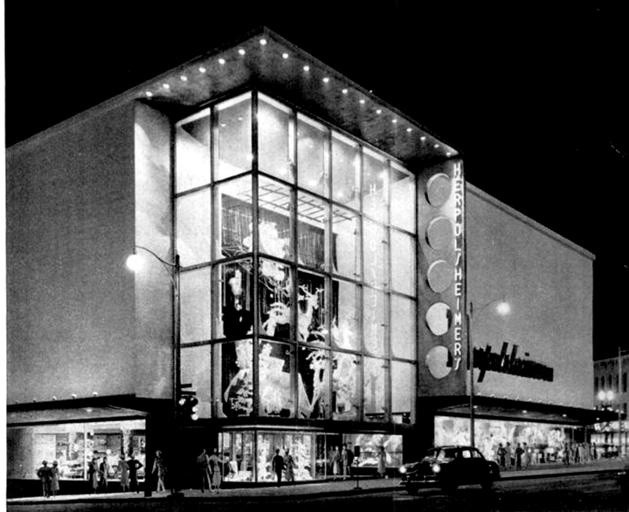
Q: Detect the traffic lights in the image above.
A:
186,398,199,421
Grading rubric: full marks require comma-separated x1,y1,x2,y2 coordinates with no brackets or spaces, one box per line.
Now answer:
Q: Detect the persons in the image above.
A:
199,449,213,493
282,449,298,485
271,448,284,487
87,453,143,494
376,446,386,477
50,461,63,495
208,448,228,491
37,459,52,498
151,450,166,493
496,441,598,471
329,443,355,481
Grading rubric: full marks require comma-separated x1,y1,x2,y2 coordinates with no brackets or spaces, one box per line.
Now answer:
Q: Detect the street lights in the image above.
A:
124,244,182,500
468,292,514,447
598,388,614,459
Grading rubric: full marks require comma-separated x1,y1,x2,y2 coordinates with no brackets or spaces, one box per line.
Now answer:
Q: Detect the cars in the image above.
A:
398,446,501,494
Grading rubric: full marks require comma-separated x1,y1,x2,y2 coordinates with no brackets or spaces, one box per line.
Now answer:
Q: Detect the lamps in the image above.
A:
469,294,512,320
124,244,179,291
